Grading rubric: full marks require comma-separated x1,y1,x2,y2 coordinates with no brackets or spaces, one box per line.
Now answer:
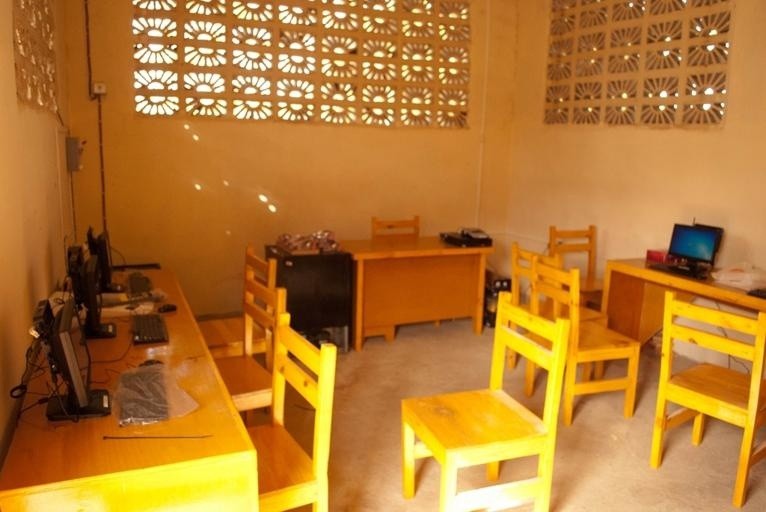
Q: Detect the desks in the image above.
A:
0,264,263,511
601,249,766,389
334,234,496,354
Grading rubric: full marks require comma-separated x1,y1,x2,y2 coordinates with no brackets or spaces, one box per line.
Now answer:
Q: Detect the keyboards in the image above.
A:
654,263,699,278
129,276,152,293
119,370,169,424
131,313,169,345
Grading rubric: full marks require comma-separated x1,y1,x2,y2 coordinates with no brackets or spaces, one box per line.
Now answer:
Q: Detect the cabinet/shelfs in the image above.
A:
267,241,353,352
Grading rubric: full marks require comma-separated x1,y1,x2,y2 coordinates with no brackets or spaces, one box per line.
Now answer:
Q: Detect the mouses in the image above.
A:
138,358,163,368
159,304,177,313
697,274,707,280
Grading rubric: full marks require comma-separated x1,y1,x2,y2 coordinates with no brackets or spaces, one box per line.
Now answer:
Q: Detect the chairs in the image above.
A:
197,245,278,366
400,288,574,512
504,239,610,397
370,213,440,330
525,252,643,426
649,288,766,509
237,310,340,511
219,263,290,421
547,222,604,305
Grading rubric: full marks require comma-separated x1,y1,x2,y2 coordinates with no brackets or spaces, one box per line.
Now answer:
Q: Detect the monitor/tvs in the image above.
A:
45,299,111,420
76,254,117,339
668,221,723,273
96,231,124,294
